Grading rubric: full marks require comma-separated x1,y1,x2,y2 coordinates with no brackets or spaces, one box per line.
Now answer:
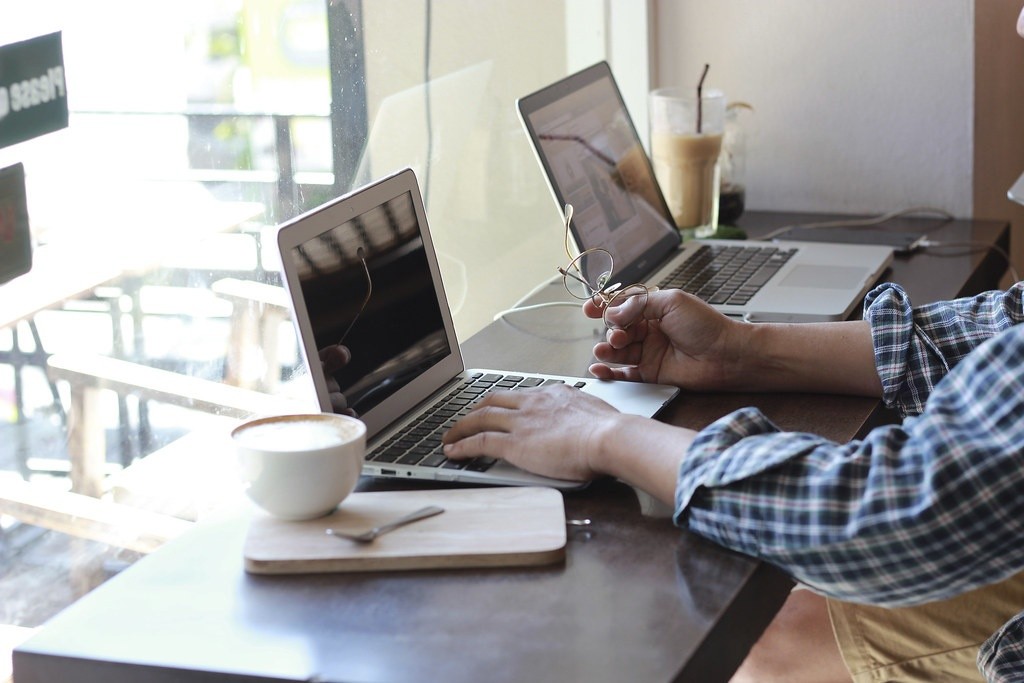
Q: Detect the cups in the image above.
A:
229,412,368,522
649,86,726,239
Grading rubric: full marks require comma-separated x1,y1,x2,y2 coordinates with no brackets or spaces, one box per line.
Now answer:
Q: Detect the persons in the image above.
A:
442,282,1024,683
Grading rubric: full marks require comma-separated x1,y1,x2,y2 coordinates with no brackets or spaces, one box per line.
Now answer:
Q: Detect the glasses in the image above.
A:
552,202,657,333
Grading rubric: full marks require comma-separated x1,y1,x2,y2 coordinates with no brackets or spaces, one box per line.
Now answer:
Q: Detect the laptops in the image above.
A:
519,59,893,324
272,169,680,490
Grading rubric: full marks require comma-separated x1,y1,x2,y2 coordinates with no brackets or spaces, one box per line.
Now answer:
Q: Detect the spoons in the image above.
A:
326,506,445,542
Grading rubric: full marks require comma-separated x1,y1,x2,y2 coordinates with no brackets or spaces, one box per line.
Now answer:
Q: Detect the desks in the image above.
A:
9,200,1012,683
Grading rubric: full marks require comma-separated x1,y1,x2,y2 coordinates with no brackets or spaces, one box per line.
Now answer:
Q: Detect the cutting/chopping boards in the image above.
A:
242,488,568,574
